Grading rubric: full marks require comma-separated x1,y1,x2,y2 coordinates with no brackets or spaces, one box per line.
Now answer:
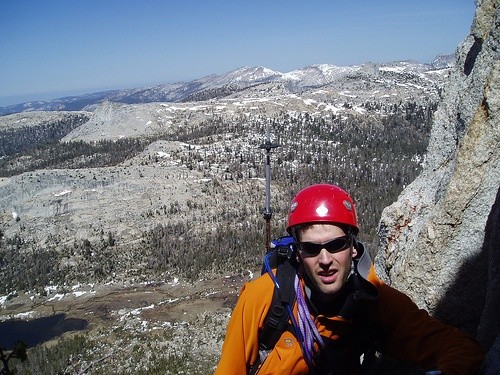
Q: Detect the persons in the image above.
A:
213,184,485,375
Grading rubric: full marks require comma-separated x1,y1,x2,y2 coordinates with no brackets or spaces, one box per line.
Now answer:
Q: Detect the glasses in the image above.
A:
296,235,355,253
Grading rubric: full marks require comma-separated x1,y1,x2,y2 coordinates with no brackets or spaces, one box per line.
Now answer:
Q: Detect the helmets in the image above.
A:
286,183,360,232
